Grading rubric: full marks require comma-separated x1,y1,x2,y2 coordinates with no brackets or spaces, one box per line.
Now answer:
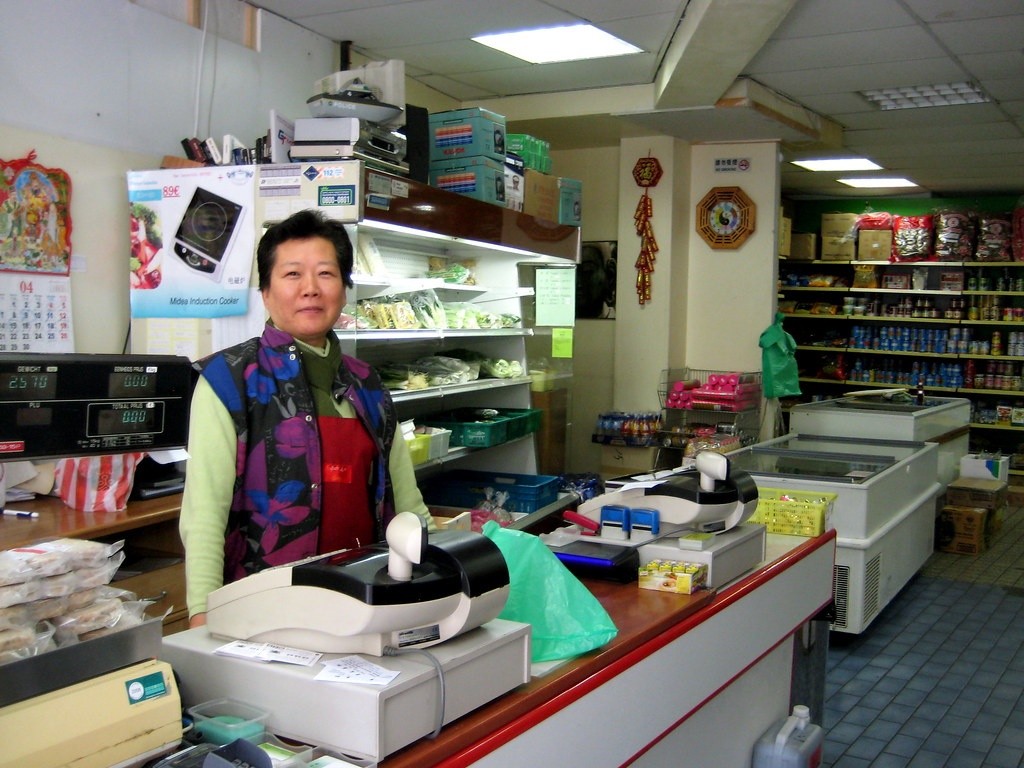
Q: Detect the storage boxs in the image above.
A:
447,407,542,441
523,168,560,223
415,469,559,513
429,155,506,207
638,560,708,595
560,178,582,227
938,454,1024,556
417,410,513,448
428,107,507,161
507,132,553,175
505,150,524,213
413,426,452,460
400,434,429,464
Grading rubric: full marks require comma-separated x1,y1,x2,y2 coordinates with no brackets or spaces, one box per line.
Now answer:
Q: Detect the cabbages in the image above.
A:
378,294,445,330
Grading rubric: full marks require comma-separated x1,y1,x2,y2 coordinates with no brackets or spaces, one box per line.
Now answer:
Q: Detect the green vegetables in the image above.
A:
378,346,523,389
443,303,521,330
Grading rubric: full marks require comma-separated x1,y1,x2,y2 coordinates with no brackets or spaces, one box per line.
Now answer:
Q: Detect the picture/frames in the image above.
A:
575,240,617,320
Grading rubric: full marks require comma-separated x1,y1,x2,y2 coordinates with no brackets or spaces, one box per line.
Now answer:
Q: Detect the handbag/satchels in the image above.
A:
54,453,146,512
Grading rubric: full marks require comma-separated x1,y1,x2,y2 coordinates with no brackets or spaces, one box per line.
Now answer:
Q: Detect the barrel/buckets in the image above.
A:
752,704,823,768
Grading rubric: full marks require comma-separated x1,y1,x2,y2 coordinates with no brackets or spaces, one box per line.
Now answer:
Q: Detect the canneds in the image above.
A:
663,421,755,448
849,278,1024,392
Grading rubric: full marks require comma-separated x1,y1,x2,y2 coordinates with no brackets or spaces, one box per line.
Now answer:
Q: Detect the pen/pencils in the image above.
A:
0,510,39,518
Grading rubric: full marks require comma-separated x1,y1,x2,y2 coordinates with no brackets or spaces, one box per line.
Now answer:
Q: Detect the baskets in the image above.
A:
420,405,542,448
419,469,560,514
402,423,452,465
745,487,839,538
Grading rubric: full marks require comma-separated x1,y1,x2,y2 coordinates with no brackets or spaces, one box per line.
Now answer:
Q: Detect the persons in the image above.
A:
180,210,437,628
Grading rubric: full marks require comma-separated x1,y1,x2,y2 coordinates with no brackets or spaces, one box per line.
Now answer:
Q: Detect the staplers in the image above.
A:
562,510,600,536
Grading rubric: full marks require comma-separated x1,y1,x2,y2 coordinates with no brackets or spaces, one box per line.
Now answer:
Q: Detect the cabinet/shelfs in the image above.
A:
0,492,190,639
333,274,534,474
653,367,763,469
779,260,1024,475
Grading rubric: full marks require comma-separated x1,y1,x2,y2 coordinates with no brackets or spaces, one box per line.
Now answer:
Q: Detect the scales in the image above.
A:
0,350,196,768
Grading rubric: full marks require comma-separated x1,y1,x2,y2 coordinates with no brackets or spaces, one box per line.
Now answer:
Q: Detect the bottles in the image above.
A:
595,411,662,437
912,296,967,319
